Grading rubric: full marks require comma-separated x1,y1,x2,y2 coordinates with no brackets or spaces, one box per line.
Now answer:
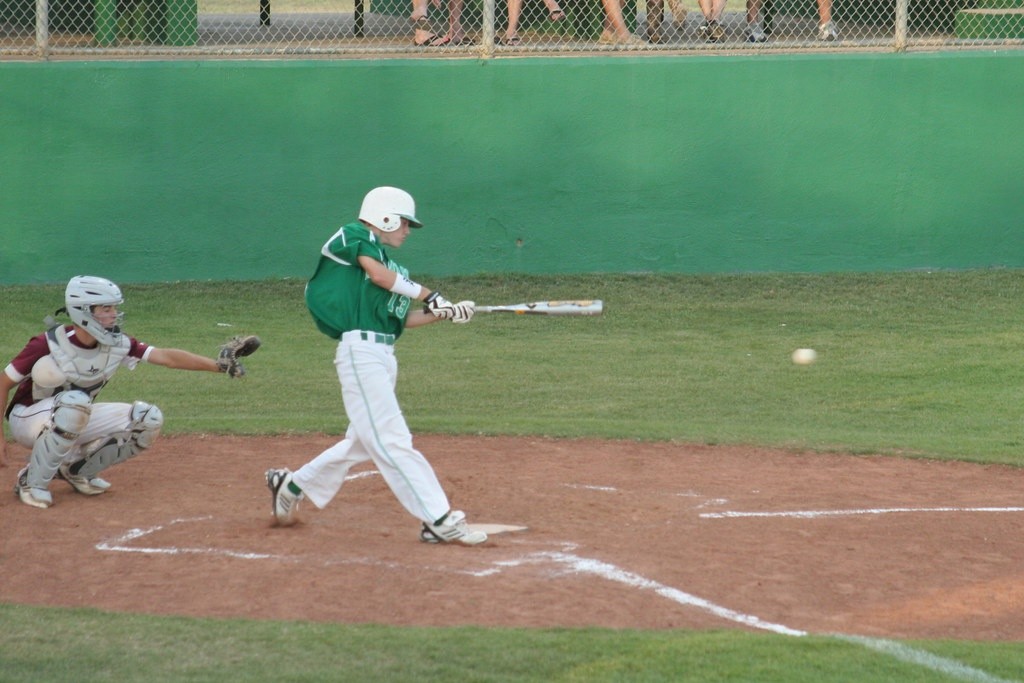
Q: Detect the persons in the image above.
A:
408,0,839,47
264,186,489,546
0,274,263,509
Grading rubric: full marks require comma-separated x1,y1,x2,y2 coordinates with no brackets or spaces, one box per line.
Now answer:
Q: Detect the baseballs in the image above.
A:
792,348,809,364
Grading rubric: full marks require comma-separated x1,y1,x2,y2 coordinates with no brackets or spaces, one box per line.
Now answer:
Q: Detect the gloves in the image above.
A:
451,301,476,323
423,292,456,320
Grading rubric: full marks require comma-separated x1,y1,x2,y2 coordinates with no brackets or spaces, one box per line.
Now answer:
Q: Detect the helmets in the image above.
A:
65,275,124,346
359,187,423,232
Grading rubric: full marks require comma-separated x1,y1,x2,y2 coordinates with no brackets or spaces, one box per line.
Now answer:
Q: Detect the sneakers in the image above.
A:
265,468,304,525
421,510,487,544
16,468,48,508
58,464,104,494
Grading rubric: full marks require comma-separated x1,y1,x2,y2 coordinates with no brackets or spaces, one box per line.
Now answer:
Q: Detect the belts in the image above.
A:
339,332,394,344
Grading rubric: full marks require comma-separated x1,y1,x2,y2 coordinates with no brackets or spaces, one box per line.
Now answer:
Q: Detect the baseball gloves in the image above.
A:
215,334,262,380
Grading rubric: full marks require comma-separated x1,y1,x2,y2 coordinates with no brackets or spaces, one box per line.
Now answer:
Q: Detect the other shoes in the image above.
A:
550,10,565,21
413,19,437,32
703,19,725,42
598,32,646,47
505,37,520,47
818,22,839,42
744,24,768,44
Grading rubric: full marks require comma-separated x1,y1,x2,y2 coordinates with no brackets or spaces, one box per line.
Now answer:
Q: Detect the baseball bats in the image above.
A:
422,299,603,315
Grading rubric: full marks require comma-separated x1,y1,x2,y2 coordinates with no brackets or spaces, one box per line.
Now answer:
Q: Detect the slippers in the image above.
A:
451,37,475,47
413,34,450,47
